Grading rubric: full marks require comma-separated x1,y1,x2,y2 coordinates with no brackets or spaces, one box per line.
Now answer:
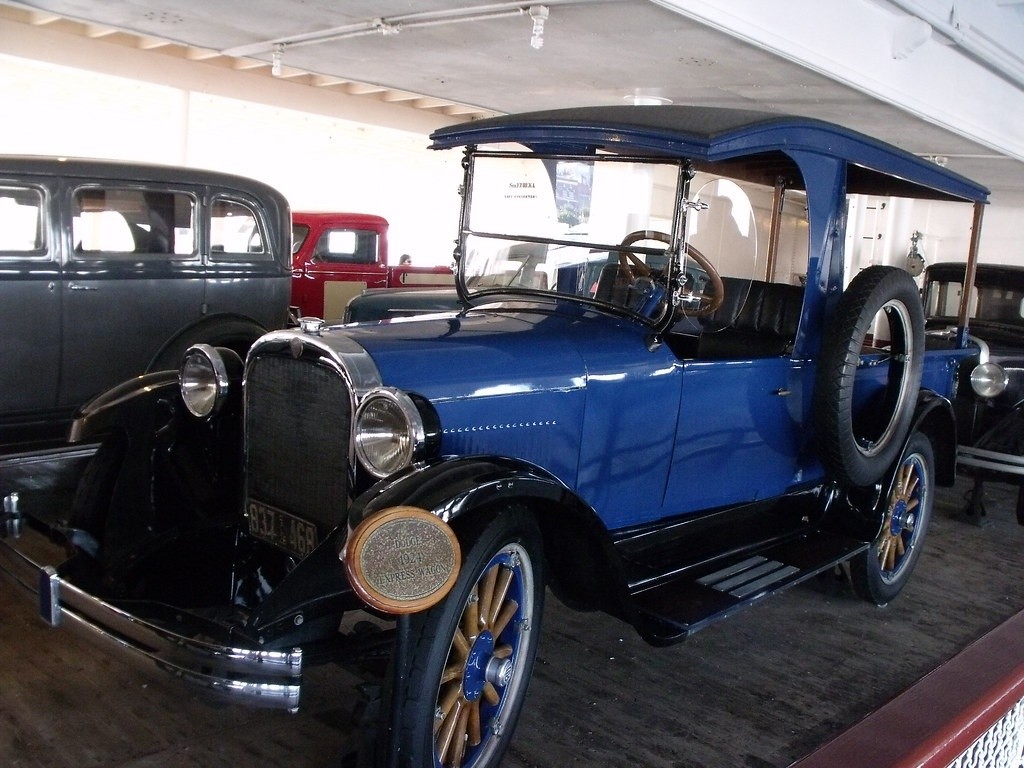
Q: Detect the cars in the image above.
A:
1,103,987,768
896,262,1024,527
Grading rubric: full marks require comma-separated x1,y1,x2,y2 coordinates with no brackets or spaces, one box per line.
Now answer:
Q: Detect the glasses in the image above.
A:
405,261,412,263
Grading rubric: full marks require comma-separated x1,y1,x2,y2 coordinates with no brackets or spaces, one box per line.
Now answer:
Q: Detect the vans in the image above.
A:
1,152,293,462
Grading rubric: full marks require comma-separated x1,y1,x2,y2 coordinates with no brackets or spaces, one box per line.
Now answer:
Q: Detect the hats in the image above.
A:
399,254,409,265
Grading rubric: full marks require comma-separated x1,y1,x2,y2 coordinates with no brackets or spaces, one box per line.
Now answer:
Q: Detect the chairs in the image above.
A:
694,275,805,358
136,229,169,254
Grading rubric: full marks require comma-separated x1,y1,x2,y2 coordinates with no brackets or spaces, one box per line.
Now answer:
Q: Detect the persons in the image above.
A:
399,254,412,266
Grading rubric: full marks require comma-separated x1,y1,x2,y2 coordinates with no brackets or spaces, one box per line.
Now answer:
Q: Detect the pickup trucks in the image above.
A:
243,212,460,330
